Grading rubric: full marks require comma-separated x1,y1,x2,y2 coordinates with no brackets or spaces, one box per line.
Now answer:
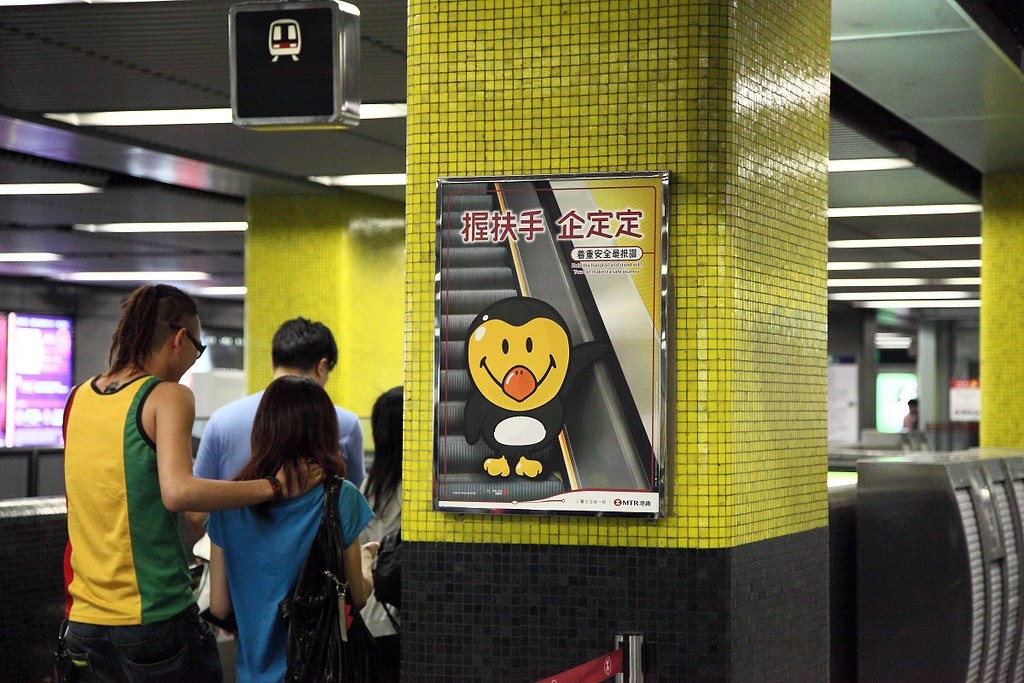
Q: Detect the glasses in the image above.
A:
167,322,209,360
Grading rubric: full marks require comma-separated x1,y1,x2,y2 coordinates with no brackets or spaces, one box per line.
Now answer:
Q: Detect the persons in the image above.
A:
62,285,328,683
903,400,918,430
185,316,364,491
356,385,404,683
202,374,379,683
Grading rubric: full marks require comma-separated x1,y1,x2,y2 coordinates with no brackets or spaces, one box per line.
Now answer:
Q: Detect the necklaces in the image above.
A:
130,358,150,374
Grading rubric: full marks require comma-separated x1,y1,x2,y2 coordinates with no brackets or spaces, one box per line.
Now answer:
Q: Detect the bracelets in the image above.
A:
361,546,376,554
266,476,283,502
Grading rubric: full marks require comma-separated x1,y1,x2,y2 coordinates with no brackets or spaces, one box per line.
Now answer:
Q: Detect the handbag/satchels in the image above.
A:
281,475,379,683
372,525,401,637
